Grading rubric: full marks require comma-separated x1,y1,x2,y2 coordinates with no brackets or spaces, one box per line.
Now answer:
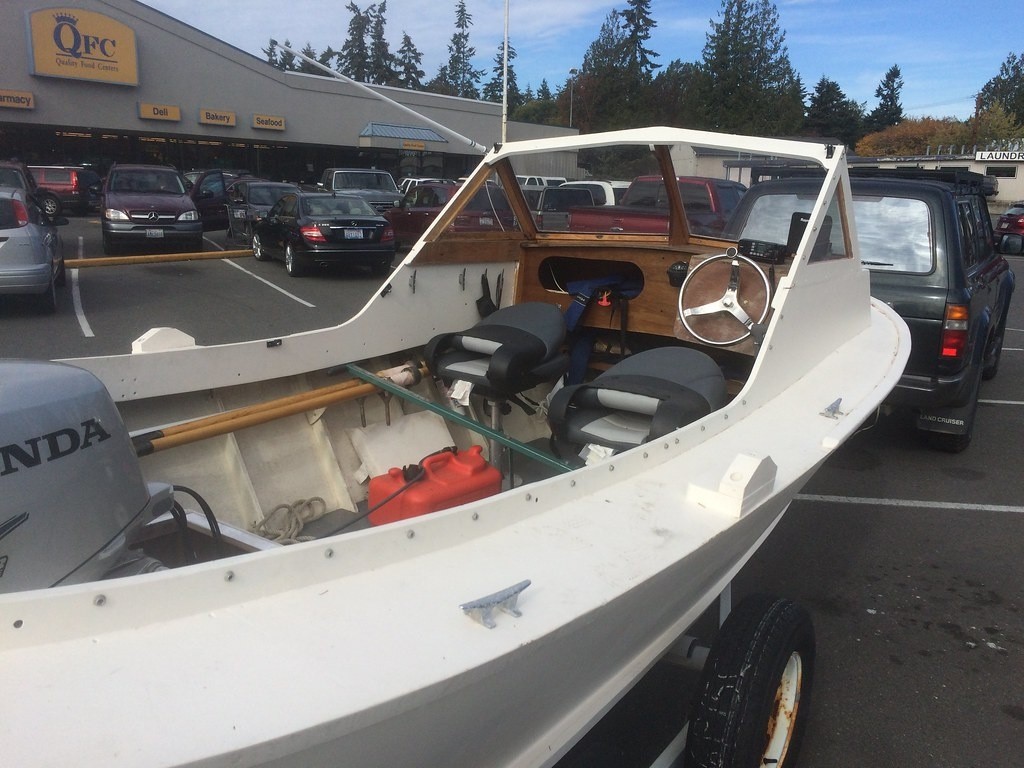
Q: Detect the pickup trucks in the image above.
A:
565,174,748,238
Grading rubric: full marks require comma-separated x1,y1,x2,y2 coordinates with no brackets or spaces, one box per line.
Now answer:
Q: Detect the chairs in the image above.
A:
544,347,726,450
311,208,362,215
425,302,572,493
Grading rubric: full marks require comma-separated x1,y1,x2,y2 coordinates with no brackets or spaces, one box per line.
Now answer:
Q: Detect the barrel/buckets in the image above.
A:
370,444,503,527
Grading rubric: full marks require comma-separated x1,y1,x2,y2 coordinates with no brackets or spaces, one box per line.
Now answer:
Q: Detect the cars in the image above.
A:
0,188,70,315
396,175,567,209
252,192,397,276
0,157,48,215
519,184,595,232
995,201,1024,256
559,181,632,207
160,170,258,231
316,167,405,216
226,183,311,248
89,161,231,256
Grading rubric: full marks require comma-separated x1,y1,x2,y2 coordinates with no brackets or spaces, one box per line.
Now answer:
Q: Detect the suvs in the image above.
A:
383,182,515,260
26,165,102,216
724,176,1016,455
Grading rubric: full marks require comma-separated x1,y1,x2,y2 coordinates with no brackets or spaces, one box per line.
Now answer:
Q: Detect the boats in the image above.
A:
1,125,913,768
